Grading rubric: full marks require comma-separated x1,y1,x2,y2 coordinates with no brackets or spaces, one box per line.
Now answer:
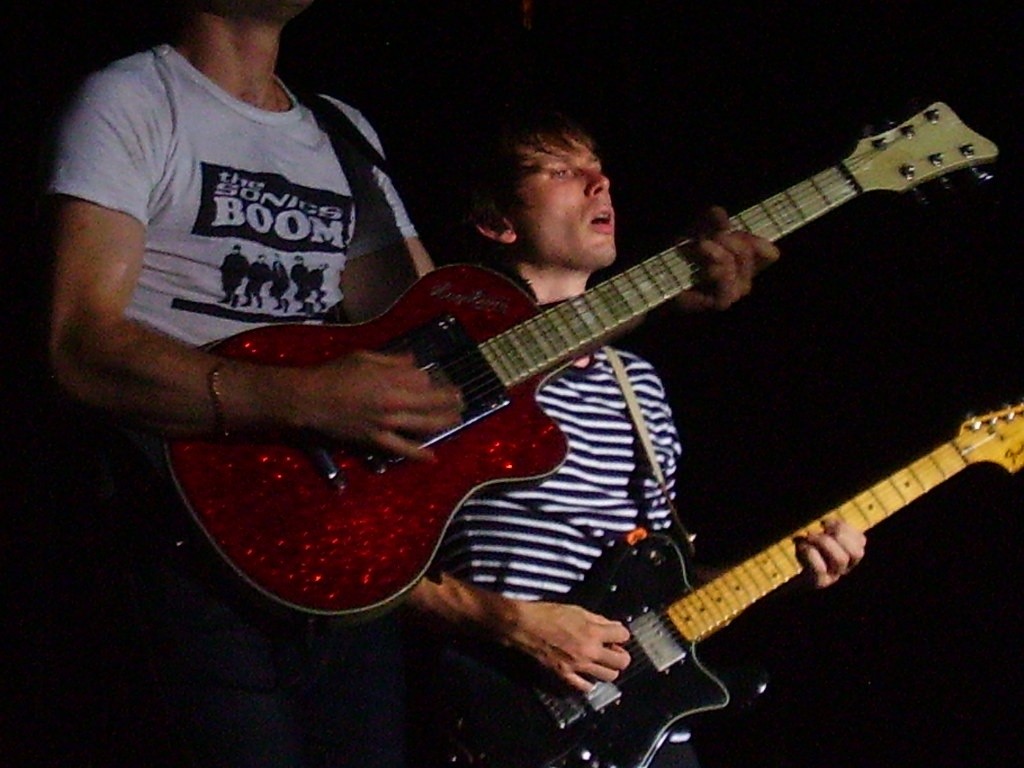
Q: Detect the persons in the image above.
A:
401,106,870,768
42,0,437,768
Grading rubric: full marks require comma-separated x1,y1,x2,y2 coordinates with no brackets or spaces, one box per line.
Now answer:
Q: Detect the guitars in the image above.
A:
162,98,1010,615
391,397,1024,766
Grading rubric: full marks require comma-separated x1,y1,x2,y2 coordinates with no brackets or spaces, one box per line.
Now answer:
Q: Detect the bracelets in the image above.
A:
206,351,233,439
